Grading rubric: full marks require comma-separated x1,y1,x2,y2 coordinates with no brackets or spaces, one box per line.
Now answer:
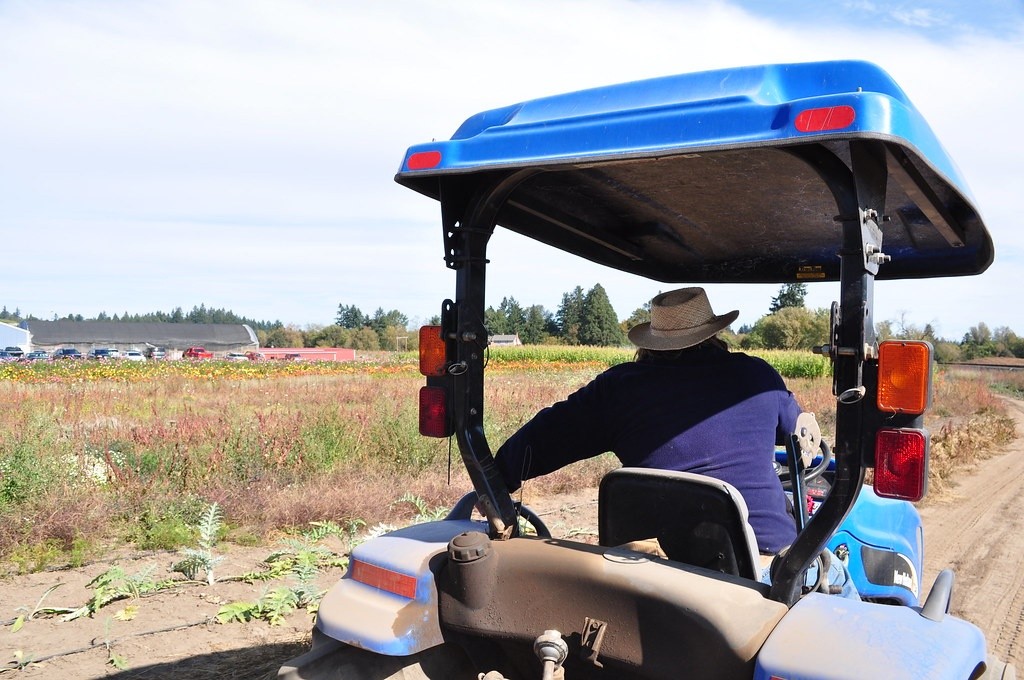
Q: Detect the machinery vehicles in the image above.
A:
274,61,996,679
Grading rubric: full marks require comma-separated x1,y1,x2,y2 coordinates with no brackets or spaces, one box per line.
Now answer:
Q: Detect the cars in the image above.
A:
142,346,168,361
51,347,83,360
0,347,50,362
87,349,112,362
225,351,266,363
108,348,147,363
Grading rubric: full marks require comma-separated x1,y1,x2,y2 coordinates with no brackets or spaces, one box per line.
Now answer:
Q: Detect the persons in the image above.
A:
472,288,865,603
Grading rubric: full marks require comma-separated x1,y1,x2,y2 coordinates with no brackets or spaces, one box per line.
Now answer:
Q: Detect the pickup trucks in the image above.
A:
180,347,212,360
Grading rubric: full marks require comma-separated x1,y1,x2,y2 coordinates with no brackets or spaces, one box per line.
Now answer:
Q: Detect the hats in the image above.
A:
628,288,740,351
